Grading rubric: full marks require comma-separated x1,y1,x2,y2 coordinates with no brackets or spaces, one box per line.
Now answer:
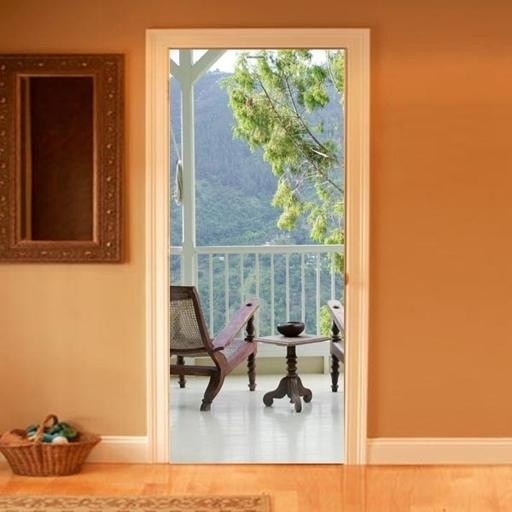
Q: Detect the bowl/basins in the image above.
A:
277,322,304,338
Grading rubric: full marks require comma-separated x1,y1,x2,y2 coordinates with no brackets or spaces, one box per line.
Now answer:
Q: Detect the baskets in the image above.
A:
0,414,102,477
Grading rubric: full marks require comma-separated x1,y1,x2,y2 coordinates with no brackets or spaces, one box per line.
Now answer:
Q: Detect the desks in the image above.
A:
251,332,332,413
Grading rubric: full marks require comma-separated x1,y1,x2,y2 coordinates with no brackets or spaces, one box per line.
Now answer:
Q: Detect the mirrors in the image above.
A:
1,51,125,264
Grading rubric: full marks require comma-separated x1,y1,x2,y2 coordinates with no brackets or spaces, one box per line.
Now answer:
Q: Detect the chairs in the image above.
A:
169,282,261,412
326,297,345,393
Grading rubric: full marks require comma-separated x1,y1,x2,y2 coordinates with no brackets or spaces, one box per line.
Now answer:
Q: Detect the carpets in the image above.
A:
0,493,274,512
